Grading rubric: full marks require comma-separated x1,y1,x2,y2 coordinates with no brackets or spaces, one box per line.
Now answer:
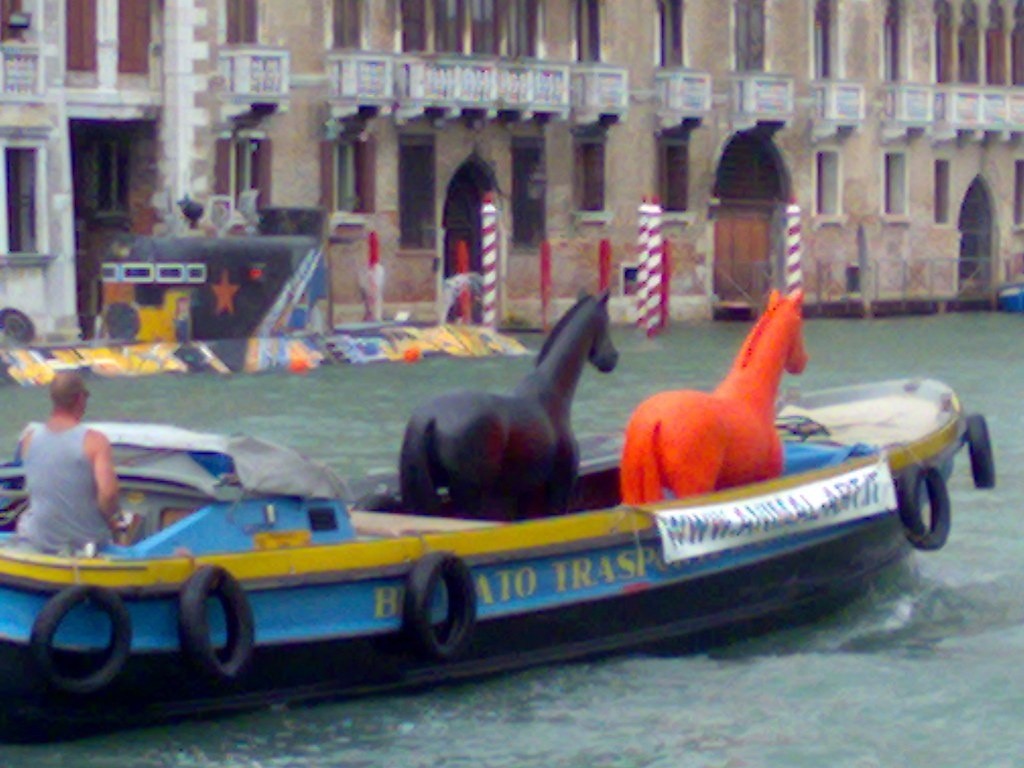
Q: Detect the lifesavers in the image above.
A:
173,564,256,675
30,581,128,698
399,552,477,655
898,461,955,552
966,411,996,489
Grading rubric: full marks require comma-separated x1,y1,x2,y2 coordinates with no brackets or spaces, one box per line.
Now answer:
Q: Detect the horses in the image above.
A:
399,286,620,524
619,286,809,505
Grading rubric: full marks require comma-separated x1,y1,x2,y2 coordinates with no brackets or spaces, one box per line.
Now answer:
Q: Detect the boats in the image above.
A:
0,378,994,747
0,230,526,383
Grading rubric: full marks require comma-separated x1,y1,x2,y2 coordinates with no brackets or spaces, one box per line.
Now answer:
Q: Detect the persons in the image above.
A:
16,369,118,554
